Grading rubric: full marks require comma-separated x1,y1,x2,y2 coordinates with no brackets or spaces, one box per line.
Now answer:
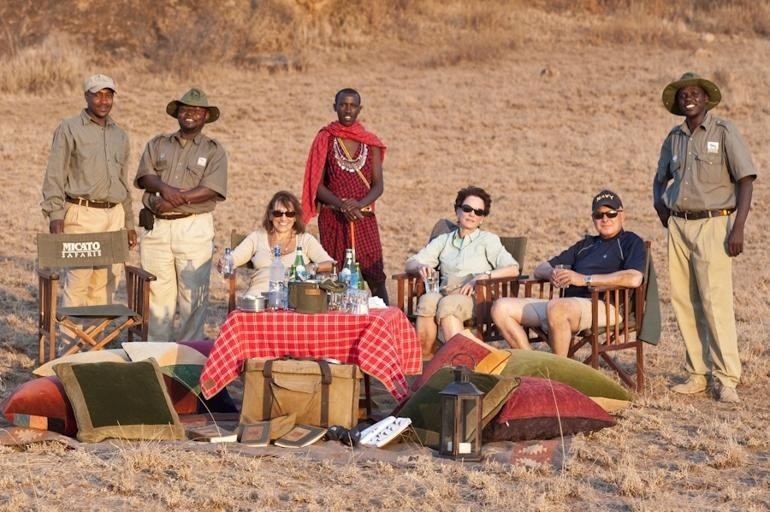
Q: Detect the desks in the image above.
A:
198,304,423,419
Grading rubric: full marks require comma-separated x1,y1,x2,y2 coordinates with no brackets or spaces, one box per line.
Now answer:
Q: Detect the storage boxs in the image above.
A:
239,357,365,435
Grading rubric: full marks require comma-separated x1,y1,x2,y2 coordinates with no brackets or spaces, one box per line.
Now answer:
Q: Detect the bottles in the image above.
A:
328,261,338,281
267,246,285,308
221,246,234,278
342,247,364,289
288,242,307,282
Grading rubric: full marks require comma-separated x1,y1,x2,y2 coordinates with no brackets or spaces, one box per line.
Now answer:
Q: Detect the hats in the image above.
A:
84,74,117,93
592,190,623,210
167,89,220,123
663,73,721,115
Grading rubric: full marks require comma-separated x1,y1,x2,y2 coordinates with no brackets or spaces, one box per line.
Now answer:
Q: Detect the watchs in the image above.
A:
584,274,592,289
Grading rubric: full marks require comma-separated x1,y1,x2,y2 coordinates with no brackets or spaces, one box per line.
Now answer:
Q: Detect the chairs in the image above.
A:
35,228,158,365
226,230,255,318
391,220,527,344
519,235,651,394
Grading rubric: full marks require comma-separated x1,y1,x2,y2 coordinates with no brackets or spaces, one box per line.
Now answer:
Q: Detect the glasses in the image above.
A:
594,210,621,219
272,210,295,217
462,205,485,215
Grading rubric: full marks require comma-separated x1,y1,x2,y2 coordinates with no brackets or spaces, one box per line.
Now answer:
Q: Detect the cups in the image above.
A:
236,291,272,312
554,262,573,289
423,269,440,293
328,289,369,314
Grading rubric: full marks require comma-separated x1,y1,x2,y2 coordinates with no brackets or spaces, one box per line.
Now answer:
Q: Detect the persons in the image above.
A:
300,88,389,306
40,74,137,358
217,190,338,295
652,72,757,403
490,190,646,357
133,88,227,342
404,186,520,362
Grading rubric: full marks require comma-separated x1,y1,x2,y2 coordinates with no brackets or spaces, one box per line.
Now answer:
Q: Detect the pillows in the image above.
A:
411,329,511,394
500,349,634,404
483,376,618,446
392,363,520,451
33,349,130,381
49,359,187,443
1,377,77,438
121,340,209,369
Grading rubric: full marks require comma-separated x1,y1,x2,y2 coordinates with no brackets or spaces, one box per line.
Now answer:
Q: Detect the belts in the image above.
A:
673,210,734,219
156,213,191,219
68,198,115,208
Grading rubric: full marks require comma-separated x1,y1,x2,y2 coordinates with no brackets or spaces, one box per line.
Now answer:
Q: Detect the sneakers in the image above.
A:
671,375,707,393
712,384,738,402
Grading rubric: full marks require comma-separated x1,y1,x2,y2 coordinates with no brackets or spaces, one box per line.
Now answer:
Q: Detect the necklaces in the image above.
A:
333,138,368,173
